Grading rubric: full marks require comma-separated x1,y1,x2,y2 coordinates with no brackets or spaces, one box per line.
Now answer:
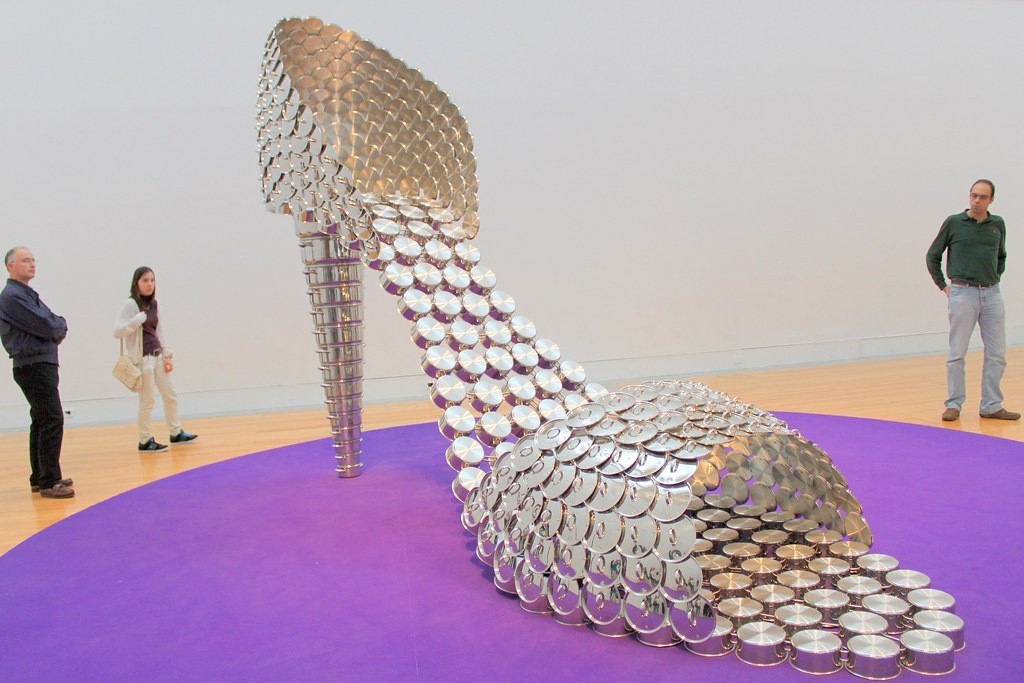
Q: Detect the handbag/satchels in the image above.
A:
113,356,143,392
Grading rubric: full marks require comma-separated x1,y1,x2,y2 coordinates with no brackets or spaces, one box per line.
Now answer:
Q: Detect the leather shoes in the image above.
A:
980,408,1021,420
31,478,73,493
942,408,960,421
40,484,74,498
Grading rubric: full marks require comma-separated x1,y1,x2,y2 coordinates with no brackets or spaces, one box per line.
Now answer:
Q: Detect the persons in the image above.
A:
925,179,1022,422
113,266,199,453
0,246,77,499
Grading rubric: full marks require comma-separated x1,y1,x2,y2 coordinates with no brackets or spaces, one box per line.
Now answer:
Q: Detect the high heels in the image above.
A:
253,15,969,682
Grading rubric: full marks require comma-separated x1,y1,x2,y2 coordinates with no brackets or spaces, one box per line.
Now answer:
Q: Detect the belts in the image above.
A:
951,279,996,287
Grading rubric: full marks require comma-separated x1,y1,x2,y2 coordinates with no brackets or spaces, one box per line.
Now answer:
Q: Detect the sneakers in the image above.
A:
137,436,168,452
170,429,198,444
